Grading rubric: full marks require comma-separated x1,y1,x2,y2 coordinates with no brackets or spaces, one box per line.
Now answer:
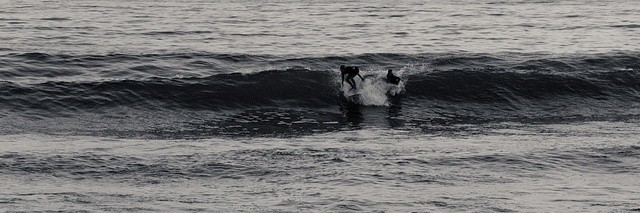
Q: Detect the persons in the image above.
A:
386,69,401,86
339,64,365,91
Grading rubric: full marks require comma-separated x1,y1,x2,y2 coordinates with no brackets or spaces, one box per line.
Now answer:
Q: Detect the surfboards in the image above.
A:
349,85,363,96
